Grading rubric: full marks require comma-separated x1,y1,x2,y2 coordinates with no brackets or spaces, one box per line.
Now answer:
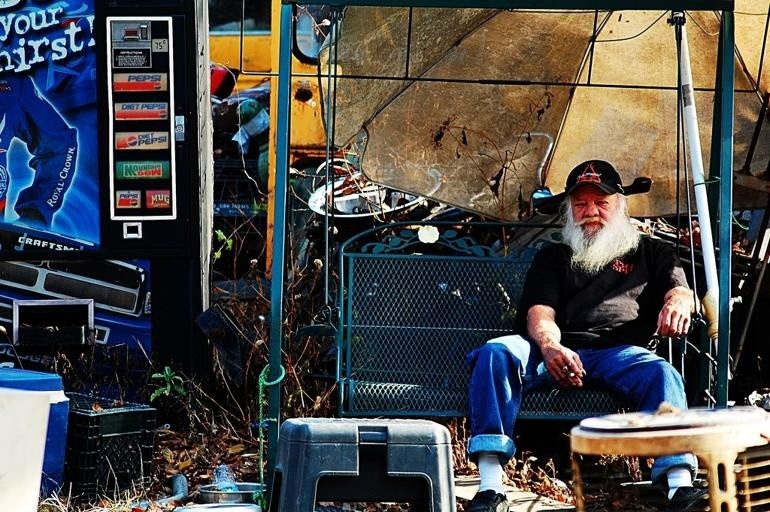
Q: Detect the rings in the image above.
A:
562,365,568,373
569,372,576,378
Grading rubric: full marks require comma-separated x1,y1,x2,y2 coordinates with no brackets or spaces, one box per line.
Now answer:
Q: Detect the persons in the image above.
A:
464,158,712,512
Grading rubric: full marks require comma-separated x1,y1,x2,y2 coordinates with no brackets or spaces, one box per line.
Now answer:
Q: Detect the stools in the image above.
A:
267,416,457,510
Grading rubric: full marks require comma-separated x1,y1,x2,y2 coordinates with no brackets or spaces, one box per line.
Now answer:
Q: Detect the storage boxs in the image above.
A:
0,367,156,511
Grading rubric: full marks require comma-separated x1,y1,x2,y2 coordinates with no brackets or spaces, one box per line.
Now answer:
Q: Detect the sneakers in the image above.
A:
464,489,509,512
664,485,711,512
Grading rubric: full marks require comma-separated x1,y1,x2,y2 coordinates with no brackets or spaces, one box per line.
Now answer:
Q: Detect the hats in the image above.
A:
565,159,621,197
